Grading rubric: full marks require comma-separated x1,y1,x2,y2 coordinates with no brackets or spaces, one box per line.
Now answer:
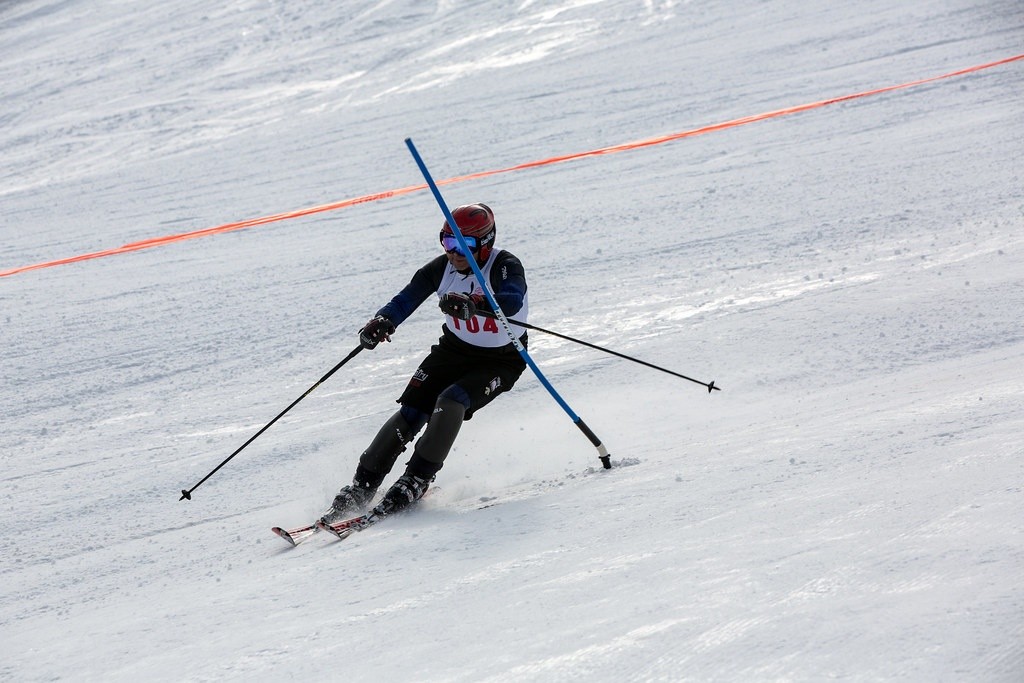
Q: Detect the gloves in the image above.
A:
358,315,395,350
439,292,485,321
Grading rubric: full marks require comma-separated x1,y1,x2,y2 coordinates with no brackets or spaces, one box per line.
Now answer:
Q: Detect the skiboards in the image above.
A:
270,483,442,548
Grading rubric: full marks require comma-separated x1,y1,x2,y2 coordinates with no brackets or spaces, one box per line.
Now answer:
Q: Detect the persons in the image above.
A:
328,204,530,516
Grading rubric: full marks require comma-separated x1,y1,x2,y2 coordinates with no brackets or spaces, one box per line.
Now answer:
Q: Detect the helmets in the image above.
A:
441,202,496,263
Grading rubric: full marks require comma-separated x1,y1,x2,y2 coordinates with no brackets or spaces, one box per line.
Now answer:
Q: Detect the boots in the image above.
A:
333,463,386,512
385,445,445,507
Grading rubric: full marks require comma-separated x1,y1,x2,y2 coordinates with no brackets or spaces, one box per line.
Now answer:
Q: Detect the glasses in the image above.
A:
440,229,482,257
